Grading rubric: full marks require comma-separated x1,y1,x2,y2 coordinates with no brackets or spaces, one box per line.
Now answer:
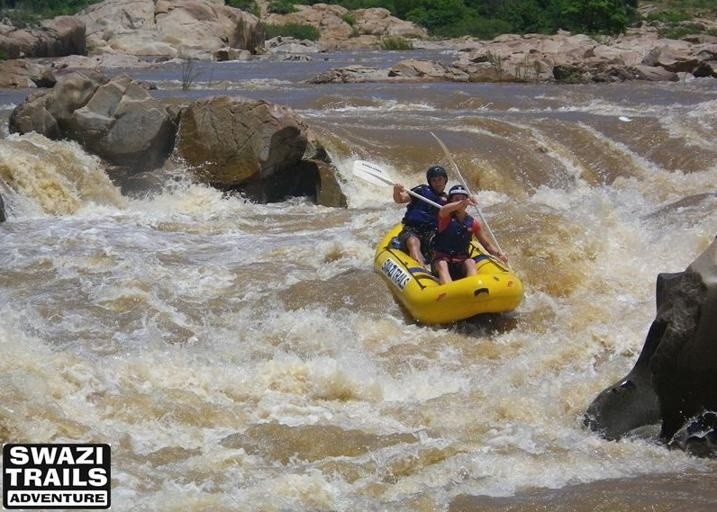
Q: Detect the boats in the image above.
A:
373,220,524,326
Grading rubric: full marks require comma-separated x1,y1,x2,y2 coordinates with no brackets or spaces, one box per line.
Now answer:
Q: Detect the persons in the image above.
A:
393,166,507,285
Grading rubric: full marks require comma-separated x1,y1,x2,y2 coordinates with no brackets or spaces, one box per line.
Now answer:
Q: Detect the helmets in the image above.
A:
426,165,448,182
449,185,468,198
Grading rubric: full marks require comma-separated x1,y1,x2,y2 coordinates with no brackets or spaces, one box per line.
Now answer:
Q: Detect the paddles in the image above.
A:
430,132,512,269
353,160,443,209
